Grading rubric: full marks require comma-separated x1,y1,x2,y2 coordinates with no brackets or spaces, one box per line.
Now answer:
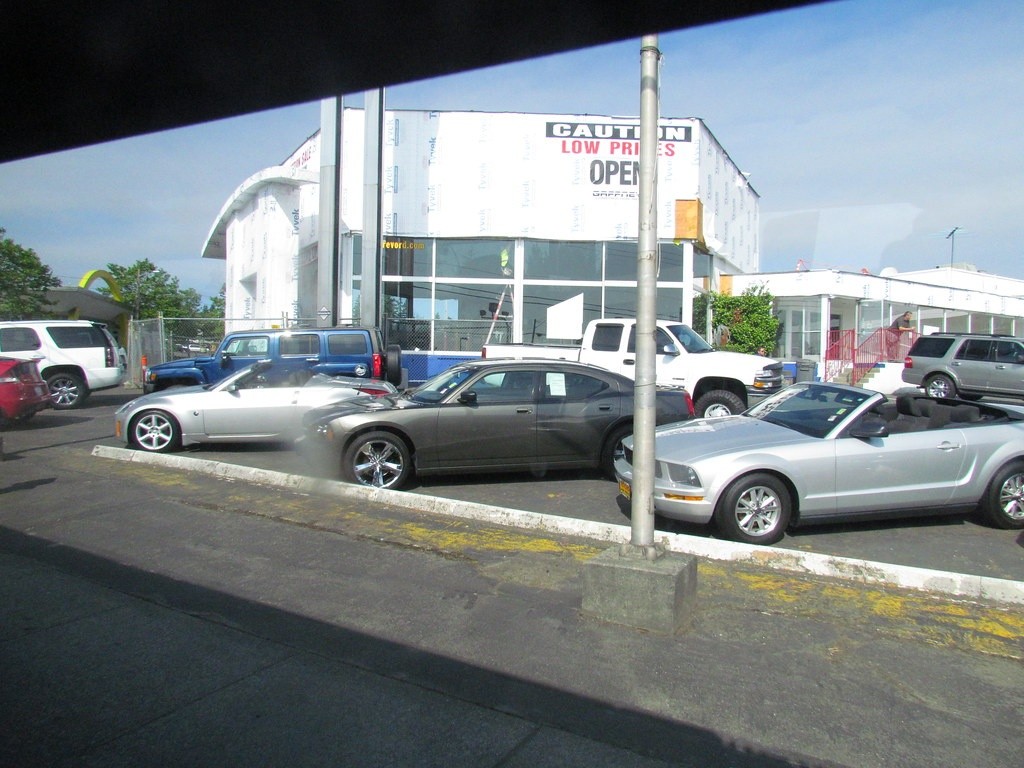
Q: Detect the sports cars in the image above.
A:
613,380,1024,546
113,359,394,455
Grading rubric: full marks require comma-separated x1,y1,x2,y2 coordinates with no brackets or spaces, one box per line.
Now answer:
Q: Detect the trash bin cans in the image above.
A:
795,359,816,384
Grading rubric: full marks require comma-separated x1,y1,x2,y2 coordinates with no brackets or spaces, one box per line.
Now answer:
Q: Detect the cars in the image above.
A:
1,353,49,427
303,357,694,509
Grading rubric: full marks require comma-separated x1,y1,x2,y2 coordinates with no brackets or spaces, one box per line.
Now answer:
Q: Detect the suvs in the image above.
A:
146,322,406,392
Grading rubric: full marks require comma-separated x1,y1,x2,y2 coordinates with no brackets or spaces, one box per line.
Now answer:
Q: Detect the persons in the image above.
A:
887,311,916,362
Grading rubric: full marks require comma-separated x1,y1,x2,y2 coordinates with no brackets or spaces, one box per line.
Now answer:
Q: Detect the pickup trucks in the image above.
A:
476,316,784,421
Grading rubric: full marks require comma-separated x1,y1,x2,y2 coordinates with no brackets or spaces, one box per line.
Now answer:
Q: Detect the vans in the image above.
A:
0,316,128,412
900,329,1024,401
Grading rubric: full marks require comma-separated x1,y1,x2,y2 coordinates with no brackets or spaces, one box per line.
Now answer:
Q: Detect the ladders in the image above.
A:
484,282,514,345
